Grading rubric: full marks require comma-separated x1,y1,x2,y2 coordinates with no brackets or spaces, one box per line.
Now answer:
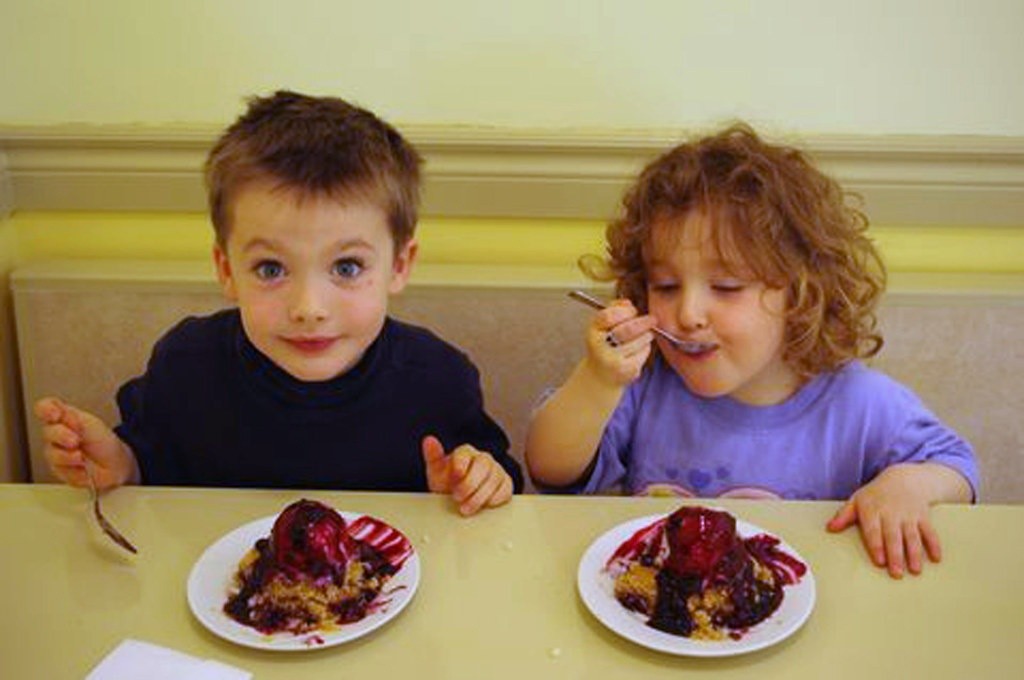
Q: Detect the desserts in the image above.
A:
226,498,396,636
614,504,785,642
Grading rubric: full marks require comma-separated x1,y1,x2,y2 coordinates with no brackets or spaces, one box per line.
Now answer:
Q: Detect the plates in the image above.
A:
186,510,423,652
576,512,817,658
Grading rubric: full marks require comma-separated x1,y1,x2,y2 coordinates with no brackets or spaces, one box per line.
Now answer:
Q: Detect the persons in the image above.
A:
525,127,980,579
33,91,524,517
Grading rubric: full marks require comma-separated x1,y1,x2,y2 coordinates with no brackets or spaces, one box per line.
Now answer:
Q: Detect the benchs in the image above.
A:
12,255,1024,507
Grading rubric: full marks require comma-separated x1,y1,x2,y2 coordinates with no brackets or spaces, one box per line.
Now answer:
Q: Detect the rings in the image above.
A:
606,333,618,347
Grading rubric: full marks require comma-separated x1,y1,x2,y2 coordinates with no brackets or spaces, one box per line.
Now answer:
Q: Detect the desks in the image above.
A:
0,482,1024,680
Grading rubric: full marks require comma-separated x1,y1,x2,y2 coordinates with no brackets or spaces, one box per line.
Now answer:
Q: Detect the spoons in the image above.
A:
53,401,139,555
566,290,720,354
